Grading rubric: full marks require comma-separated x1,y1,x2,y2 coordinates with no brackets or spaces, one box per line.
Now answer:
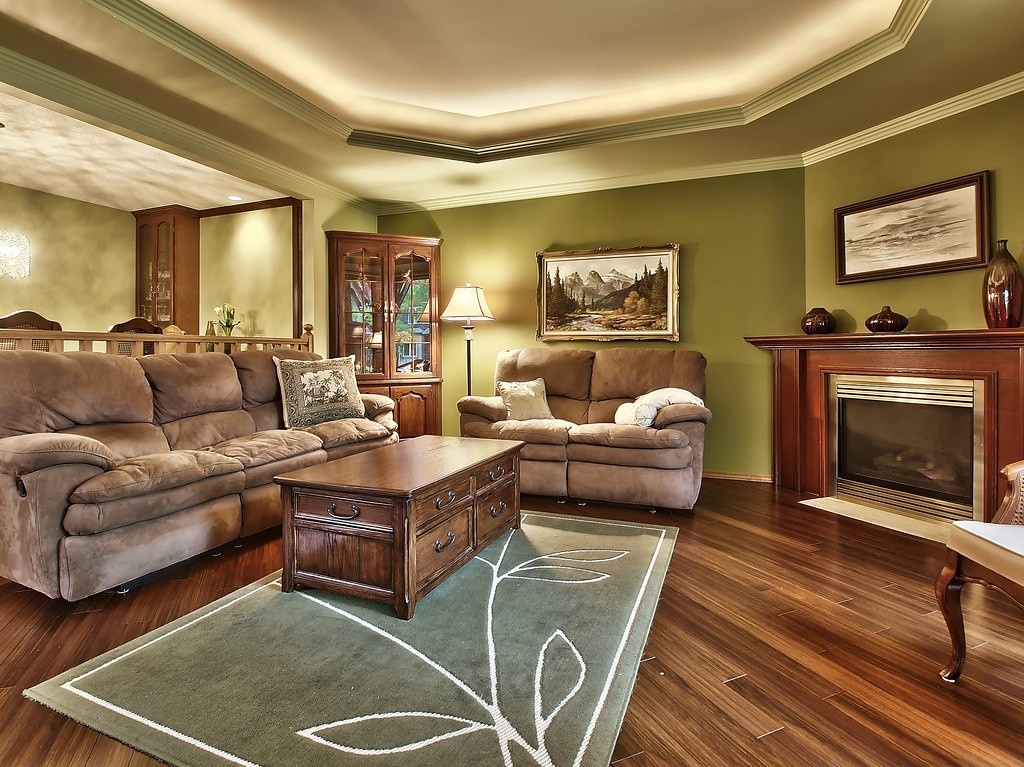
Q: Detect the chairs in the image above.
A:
163,325,188,354
0,310,62,351
111,317,163,357
934,460,1024,683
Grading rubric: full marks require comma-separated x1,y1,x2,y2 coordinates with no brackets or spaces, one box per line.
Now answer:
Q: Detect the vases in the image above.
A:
982,239,1024,328
865,306,908,332
801,307,837,335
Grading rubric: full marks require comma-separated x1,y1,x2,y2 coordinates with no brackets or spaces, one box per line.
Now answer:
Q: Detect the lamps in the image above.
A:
439,283,497,396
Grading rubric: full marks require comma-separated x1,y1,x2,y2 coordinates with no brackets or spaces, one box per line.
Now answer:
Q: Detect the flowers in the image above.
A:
214,303,241,336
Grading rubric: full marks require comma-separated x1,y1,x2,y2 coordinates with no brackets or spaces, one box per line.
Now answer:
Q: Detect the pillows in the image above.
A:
615,402,659,427
273,354,365,428
496,378,556,421
635,387,706,410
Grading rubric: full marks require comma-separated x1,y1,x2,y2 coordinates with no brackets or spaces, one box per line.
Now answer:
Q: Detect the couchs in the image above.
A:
0,348,399,602
456,348,712,514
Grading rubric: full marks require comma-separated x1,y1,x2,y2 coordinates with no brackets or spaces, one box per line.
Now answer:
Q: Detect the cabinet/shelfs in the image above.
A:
324,230,445,443
131,204,201,354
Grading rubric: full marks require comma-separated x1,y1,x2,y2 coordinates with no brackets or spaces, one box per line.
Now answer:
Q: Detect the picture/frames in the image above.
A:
534,241,682,343
833,170,993,285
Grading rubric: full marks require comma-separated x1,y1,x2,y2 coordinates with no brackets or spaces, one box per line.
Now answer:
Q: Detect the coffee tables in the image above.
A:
274,435,527,621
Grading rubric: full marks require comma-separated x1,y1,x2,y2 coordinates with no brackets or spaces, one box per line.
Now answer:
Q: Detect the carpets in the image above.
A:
29,508,681,767
